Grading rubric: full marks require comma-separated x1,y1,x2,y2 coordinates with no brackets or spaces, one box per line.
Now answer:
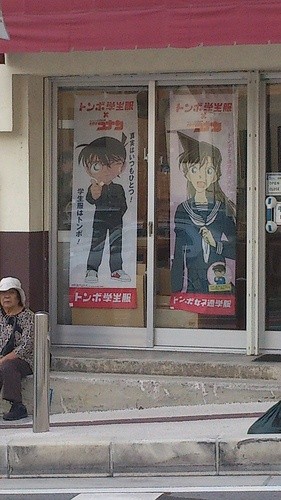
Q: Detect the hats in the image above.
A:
0,277,26,307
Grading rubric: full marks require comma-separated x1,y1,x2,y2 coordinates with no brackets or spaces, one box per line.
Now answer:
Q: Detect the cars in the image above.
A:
85,217,203,271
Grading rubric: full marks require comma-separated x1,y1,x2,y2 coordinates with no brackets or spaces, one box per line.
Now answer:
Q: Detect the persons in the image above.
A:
0,276,52,421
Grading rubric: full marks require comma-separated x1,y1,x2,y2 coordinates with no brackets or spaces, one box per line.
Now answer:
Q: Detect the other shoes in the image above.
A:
3,404,28,420
